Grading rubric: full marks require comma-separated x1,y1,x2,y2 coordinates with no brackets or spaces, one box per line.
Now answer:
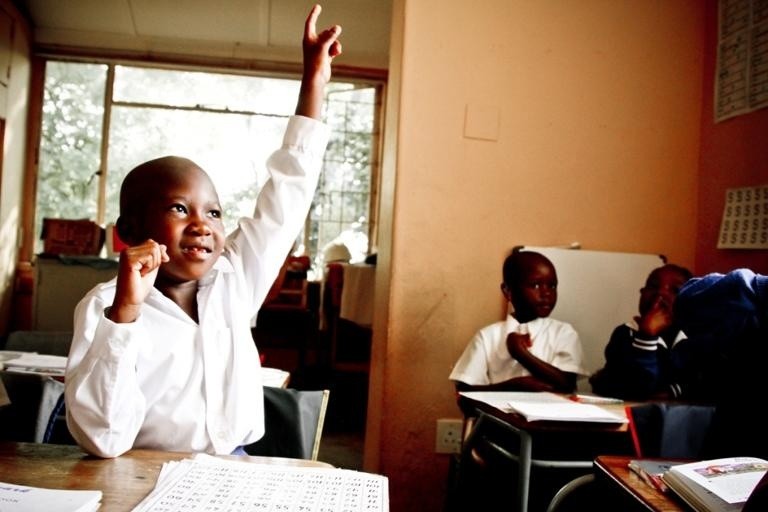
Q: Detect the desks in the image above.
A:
1,347,296,438
1,442,346,511
461,391,672,511
596,453,766,511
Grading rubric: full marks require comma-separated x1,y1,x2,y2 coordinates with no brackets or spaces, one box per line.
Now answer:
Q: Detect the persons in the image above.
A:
60,1,344,460
447,250,618,511
598,262,698,398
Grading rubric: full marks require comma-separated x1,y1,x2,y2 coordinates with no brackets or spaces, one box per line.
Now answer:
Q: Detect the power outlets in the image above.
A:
435,418,463,453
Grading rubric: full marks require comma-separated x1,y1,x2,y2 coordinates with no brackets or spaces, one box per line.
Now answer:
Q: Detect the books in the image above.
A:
659,455,768,512
627,460,687,491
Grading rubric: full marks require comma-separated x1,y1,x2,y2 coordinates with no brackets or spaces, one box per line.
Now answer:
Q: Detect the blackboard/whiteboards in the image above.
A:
505,246,667,394
